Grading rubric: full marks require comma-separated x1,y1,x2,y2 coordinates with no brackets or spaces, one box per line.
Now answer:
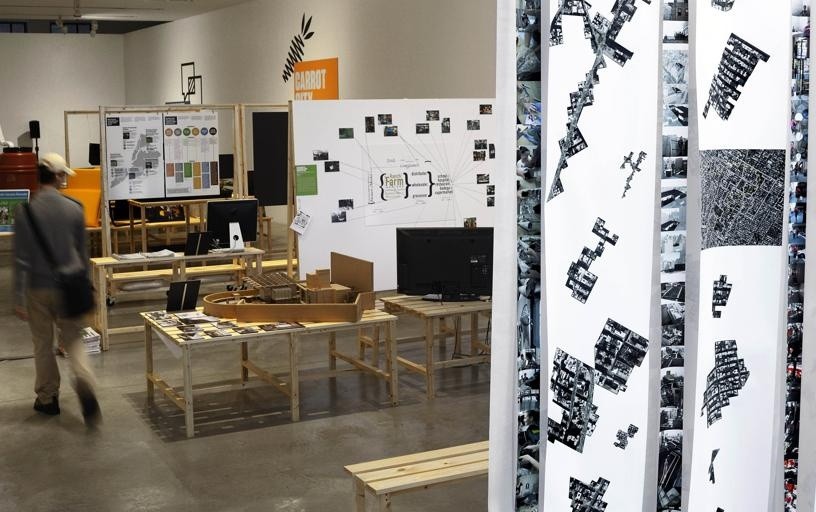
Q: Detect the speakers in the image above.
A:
29,121,40,138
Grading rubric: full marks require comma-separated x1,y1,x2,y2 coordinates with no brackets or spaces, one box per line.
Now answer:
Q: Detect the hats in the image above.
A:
39,152,77,177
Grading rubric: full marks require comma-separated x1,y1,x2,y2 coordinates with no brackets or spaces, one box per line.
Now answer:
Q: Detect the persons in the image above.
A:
8,152,105,426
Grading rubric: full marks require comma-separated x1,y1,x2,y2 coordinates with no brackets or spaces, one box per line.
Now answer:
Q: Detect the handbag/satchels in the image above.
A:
61,277,96,319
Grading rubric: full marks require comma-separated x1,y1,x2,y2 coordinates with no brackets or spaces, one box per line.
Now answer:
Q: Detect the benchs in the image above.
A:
111,215,273,255
343,441,490,512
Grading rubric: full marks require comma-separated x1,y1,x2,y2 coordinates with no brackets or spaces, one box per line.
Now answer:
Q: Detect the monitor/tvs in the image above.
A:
397,227,494,302
207,200,258,252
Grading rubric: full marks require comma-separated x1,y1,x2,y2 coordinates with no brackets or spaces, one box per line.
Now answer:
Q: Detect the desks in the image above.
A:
90,246,297,351
140,300,399,438
379,294,490,401
127,198,208,253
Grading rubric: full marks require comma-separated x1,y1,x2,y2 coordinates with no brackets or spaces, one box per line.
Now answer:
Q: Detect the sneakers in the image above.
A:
33,397,60,415
76,382,103,428
